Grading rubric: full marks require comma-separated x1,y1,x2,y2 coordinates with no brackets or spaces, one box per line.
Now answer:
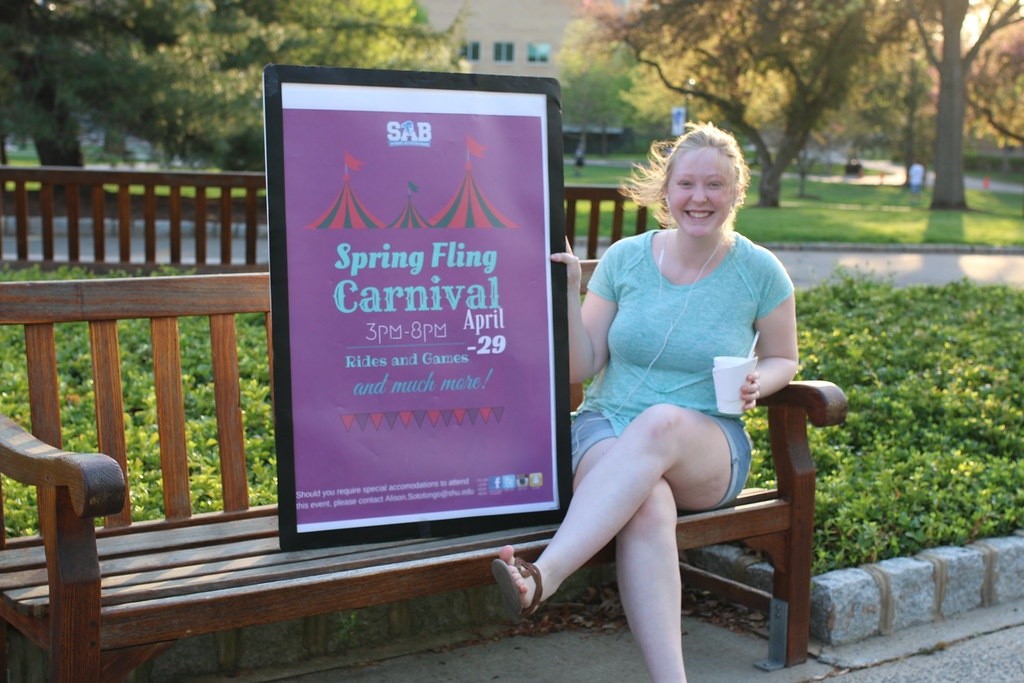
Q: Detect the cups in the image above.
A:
712,356,759,414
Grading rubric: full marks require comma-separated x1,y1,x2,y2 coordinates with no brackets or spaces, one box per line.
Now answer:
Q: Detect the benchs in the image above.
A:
0,259,852,683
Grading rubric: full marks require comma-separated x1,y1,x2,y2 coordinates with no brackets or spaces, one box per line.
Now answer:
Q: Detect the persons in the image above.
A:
572,144,586,176
907,161,926,209
841,151,864,179
490,119,800,681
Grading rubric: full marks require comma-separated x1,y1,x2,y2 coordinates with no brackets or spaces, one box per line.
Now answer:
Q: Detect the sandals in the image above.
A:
491,557,543,624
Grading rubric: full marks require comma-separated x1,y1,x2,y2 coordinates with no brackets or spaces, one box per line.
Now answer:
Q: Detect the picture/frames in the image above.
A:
261,64,574,553
